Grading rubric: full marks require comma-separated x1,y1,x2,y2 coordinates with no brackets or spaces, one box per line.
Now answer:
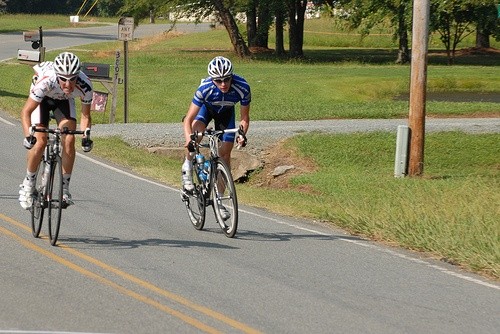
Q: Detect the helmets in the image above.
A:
207,55,234,79
54,52,80,79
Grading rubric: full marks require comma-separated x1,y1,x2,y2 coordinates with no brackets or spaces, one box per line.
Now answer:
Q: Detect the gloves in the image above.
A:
81,137,93,152
23,136,38,150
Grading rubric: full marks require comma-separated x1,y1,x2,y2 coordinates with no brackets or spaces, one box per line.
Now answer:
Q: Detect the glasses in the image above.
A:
213,78,231,83
58,76,78,82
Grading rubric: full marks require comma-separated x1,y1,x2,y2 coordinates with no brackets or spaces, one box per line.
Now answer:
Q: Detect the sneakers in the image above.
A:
62,191,74,206
181,163,195,192
18,178,36,208
217,204,231,220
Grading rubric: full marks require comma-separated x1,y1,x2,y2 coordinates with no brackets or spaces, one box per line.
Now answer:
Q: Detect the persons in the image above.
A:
19,52,92,210
181,56,251,221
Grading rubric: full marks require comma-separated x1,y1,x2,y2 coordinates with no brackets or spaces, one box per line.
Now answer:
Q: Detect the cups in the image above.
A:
196,155,205,182
201,161,210,181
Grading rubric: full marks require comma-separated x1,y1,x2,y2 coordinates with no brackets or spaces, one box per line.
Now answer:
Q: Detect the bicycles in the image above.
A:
180,125,248,238
28,125,90,246
